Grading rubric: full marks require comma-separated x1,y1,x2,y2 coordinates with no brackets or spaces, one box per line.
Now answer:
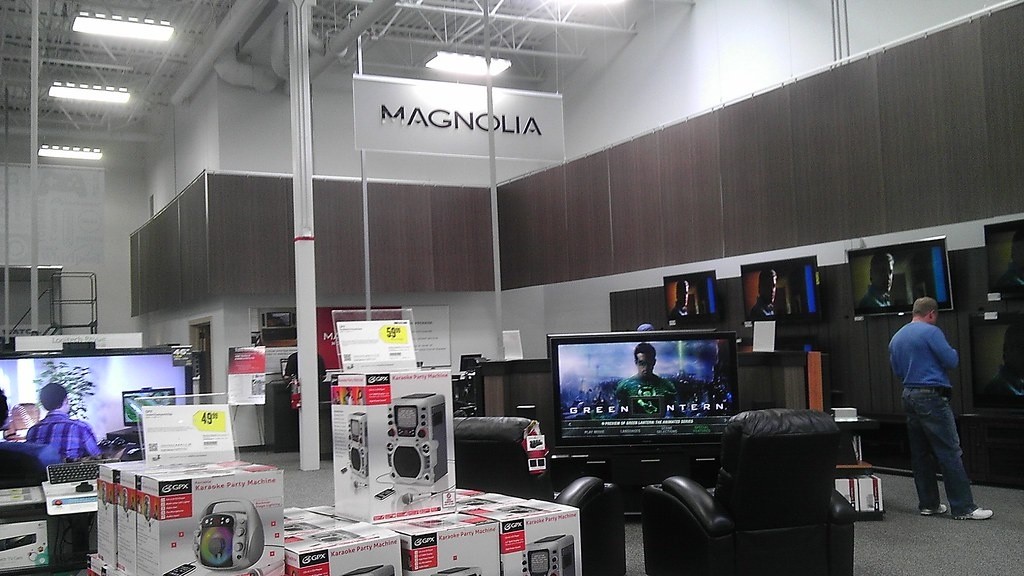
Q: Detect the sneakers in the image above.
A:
953,507,993,520
920,503,947,515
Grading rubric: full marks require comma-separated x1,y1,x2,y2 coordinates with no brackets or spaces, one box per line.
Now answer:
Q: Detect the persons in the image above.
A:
27,384,125,461
862,253,895,309
613,344,684,417
889,297,995,520
998,230,1024,286
749,270,777,316
979,325,1024,409
670,279,689,317
285,350,328,384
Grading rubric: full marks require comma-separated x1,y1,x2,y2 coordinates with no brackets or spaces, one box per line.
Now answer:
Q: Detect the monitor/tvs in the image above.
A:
984,219,1024,300
459,354,482,372
122,388,176,433
663,270,720,326
741,255,822,326
848,235,953,317
546,330,739,448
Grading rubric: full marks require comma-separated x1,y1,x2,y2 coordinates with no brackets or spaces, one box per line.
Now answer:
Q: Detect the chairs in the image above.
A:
453,416,626,576
642,408,858,576
0,442,89,555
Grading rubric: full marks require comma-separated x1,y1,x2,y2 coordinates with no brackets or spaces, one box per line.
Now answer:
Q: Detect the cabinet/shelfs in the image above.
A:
863,407,1023,488
550,418,878,521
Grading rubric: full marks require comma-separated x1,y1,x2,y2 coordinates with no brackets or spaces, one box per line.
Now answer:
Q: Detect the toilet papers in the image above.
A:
503,330,523,361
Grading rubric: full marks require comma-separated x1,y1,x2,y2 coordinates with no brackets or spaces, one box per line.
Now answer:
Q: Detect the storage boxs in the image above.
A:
87,370,582,576
835,475,883,511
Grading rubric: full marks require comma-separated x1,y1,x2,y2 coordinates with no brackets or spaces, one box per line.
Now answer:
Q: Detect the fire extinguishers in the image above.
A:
286,375,301,409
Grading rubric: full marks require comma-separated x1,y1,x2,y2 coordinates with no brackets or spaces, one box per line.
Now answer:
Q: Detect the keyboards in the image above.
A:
46,461,120,485
